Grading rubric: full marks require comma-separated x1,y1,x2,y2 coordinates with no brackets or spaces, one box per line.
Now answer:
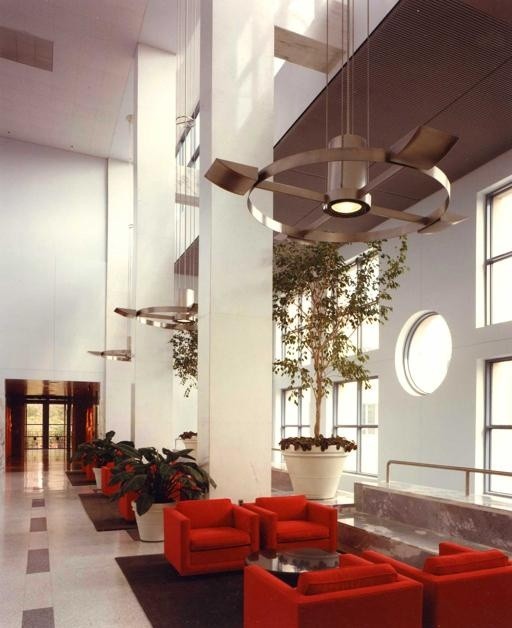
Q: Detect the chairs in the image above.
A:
163,498,261,577
243,554,424,627
256,494,337,557
100,454,200,521
362,539,511,627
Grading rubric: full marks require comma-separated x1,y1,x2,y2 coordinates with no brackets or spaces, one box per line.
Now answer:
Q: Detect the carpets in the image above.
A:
79,493,138,531
114,522,442,628
65,470,96,486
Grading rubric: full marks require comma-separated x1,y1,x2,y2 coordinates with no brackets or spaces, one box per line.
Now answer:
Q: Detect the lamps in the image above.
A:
203,1,471,243
88,115,134,362
114,1,197,330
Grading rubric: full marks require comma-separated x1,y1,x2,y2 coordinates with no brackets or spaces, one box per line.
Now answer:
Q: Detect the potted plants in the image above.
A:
167,330,198,457
68,431,134,489
272,235,410,500
